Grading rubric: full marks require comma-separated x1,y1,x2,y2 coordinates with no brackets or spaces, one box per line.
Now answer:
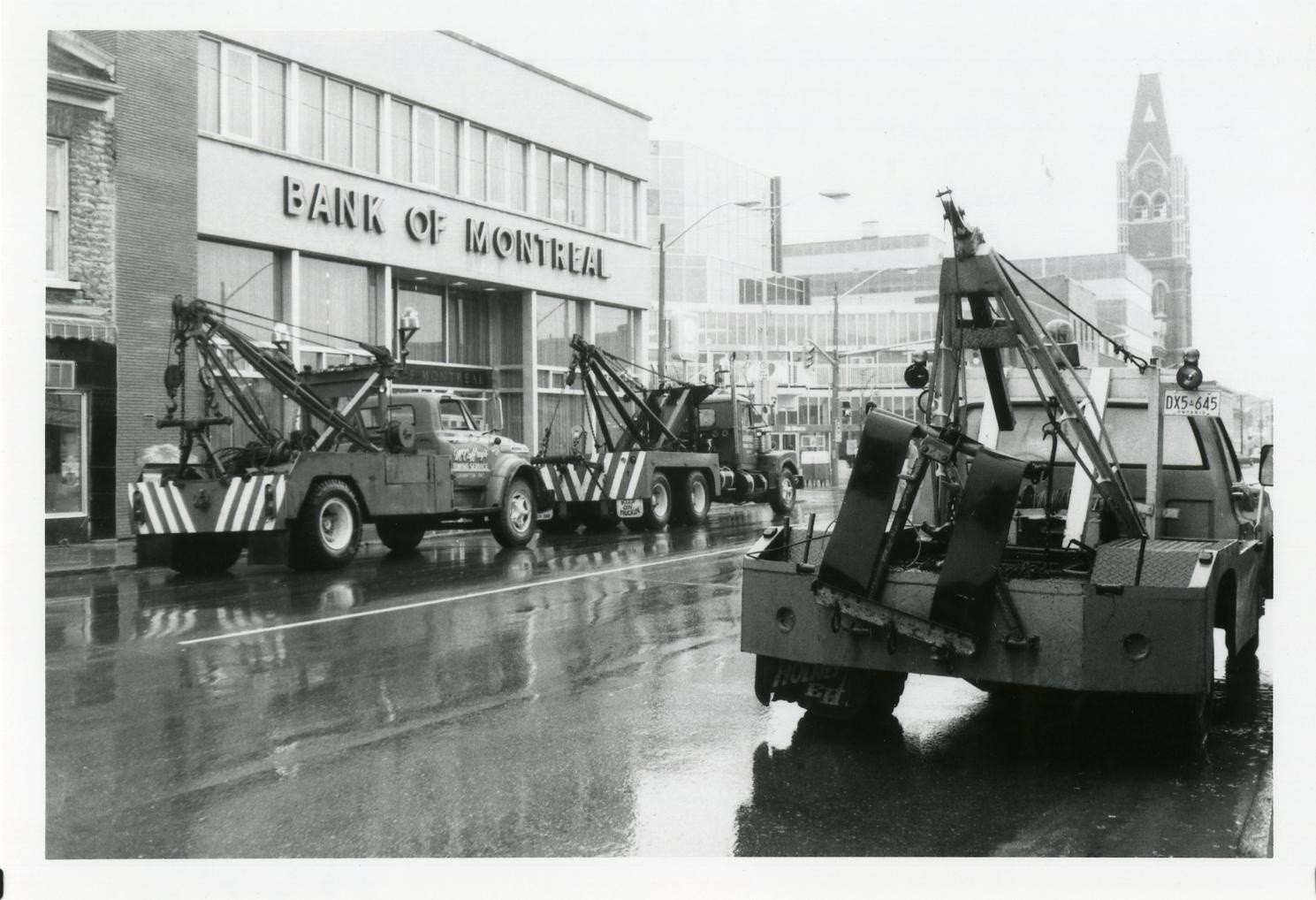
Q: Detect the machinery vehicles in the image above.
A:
127,293,556,573
516,330,805,533
740,184,1274,724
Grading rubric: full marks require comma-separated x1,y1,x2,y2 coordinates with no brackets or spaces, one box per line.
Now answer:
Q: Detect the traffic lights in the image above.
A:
804,345,814,368
842,402,851,417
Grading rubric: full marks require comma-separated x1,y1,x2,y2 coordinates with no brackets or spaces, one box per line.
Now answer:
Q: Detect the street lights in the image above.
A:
761,193,851,411
658,199,761,387
831,266,917,490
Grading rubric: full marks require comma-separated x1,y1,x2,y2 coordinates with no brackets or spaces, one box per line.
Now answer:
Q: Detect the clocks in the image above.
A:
1135,160,1166,192
